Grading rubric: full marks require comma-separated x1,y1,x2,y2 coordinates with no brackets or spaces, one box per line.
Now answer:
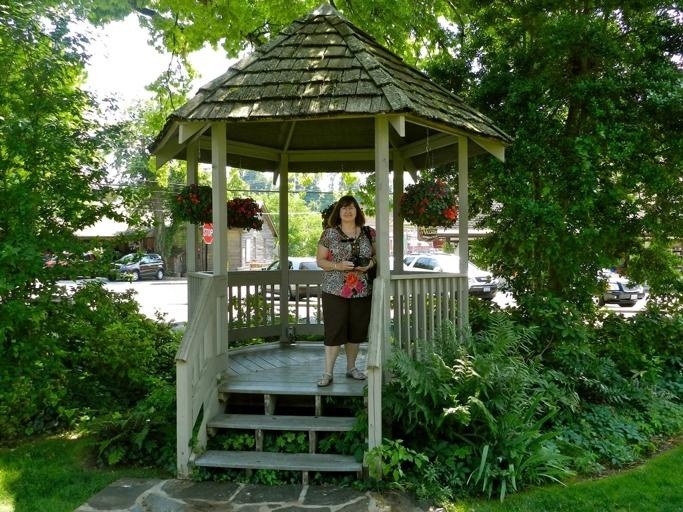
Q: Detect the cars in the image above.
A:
591,269,645,307
108,253,166,281
259,256,323,301
403,254,497,301
45,252,96,280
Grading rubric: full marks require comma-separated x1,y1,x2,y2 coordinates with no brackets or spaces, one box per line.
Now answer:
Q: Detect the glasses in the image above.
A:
339,230,357,243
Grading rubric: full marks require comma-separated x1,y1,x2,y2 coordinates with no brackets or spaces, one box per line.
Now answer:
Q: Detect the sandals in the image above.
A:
316,372,333,387
343,367,367,381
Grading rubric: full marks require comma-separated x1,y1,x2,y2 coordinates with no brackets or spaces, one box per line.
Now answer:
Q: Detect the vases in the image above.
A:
416,224,437,240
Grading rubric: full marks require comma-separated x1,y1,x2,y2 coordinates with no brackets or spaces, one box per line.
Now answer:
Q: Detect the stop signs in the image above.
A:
202,223,214,244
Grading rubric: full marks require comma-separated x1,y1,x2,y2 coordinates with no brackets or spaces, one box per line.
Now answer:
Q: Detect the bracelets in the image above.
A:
370,255,377,263
368,256,376,266
334,263,338,272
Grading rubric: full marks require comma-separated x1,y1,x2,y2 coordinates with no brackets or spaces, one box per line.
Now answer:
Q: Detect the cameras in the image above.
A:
349,257,370,267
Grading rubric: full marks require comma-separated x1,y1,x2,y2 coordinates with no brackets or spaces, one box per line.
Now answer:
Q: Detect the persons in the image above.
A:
314,196,377,386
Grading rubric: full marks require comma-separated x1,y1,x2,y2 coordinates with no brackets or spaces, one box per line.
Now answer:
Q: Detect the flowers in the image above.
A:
173,184,263,233
399,176,459,232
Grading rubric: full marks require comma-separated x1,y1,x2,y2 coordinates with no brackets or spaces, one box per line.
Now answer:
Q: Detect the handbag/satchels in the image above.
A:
368,263,376,289
